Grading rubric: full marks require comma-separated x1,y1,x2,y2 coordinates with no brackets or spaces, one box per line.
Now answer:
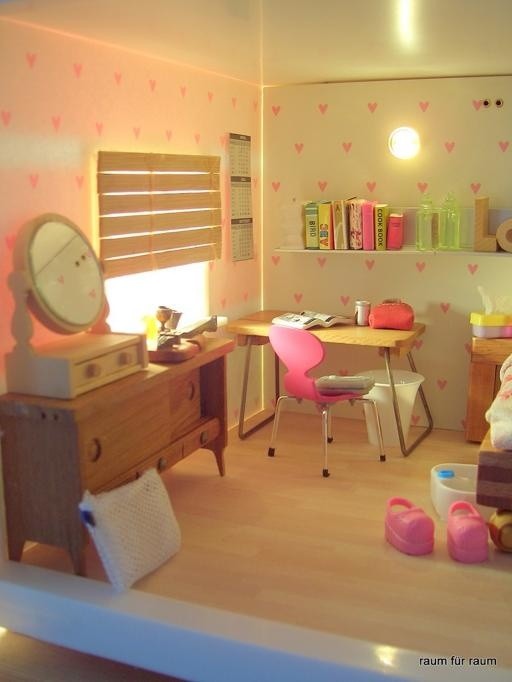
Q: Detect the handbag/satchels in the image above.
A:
369,299,414,330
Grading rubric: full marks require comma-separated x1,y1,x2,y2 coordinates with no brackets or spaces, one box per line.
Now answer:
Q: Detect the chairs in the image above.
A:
267,325,386,476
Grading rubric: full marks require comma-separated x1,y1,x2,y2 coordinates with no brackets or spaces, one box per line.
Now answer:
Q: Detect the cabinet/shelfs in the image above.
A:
0,354,229,580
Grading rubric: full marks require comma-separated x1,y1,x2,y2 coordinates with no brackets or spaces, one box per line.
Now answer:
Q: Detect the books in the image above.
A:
273,310,355,331
301,198,404,251
315,374,376,396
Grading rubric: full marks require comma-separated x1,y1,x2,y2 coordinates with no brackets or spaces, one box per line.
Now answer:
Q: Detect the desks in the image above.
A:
225,310,433,455
